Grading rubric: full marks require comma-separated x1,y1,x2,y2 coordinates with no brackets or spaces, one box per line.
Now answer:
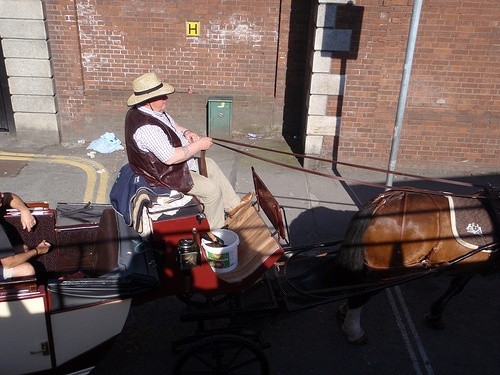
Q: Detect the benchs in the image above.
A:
125,163,285,303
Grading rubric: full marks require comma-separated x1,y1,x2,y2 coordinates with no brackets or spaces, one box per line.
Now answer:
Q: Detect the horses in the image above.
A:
334,183,500,346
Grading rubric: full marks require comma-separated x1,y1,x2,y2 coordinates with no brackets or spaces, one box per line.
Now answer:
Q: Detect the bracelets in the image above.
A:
34,247,39,256
183,130,192,138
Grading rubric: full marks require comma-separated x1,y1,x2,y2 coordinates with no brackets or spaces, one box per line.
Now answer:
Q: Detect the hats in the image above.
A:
126,72,176,106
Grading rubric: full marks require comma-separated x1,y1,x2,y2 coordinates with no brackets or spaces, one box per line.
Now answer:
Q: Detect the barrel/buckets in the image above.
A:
201,229,240,272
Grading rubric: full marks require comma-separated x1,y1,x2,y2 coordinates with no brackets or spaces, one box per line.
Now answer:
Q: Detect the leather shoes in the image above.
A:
228,192,253,219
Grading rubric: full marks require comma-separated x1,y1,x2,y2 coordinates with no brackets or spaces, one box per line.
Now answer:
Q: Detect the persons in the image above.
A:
124,72,255,232
0,192,52,281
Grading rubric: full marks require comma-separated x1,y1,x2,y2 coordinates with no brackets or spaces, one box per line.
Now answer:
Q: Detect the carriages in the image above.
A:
0,138,500,375
